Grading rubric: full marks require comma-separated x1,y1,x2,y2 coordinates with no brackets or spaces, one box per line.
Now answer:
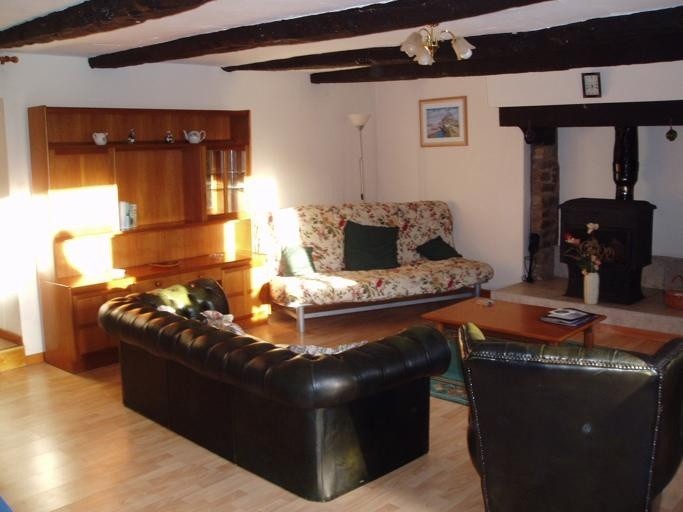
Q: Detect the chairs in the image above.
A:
457,323,683,512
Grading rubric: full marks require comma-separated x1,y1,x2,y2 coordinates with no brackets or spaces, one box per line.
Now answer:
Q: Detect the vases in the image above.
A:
583,272,600,305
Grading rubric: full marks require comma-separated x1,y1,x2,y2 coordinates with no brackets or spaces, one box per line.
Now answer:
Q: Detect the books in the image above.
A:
539,307,597,328
119,200,138,231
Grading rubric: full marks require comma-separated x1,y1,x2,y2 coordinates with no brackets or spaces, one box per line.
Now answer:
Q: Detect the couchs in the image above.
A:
97,278,451,502
254,200,493,335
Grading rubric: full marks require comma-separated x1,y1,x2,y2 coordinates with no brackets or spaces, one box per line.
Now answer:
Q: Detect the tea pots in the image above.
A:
183,129,206,143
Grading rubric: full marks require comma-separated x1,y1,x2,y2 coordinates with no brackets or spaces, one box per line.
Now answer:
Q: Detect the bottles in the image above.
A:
166,128,176,142
127,128,136,142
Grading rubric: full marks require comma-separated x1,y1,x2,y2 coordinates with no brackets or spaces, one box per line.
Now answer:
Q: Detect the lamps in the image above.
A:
347,113,370,202
400,22,476,66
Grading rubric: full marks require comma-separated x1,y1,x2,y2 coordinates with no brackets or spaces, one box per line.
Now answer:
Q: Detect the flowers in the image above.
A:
564,222,614,276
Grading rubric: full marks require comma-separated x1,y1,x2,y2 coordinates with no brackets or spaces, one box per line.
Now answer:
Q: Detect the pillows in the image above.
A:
415,236,463,260
282,246,316,277
343,219,400,269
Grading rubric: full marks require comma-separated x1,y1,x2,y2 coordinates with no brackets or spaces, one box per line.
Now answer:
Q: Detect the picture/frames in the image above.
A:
418,96,468,147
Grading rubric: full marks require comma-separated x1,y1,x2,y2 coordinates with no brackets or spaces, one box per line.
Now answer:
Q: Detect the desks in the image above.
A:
557,198,657,305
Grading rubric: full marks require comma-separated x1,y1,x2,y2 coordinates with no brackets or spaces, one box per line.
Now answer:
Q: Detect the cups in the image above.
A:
92,132,107,145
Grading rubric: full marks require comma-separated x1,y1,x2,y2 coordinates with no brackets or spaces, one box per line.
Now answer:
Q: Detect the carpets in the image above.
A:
426,321,584,405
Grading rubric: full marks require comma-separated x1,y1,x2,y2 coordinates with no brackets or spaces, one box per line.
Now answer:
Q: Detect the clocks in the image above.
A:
581,71,601,98
